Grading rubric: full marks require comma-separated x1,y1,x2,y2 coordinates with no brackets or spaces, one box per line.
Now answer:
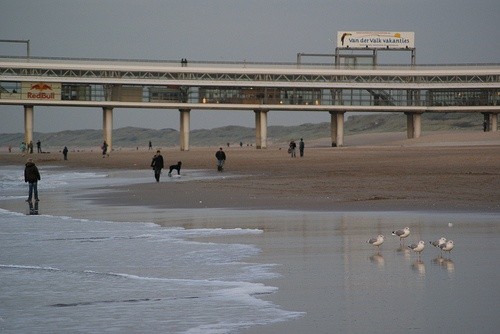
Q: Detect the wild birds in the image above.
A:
438,240,454,258
407,240,425,255
392,227,410,241
368,234,385,249
429,237,446,250
181,57,188,67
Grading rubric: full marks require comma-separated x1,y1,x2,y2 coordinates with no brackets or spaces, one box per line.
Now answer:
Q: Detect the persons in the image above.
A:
36,139,42,153
101,140,110,159
20,141,26,157
216,147,226,172
300,138,304,157
151,150,163,182
289,141,297,158
24,158,41,202
28,140,34,154
63,146,68,160
27,200,40,215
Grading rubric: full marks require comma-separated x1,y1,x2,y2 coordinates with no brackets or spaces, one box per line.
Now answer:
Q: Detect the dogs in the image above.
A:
168,161,182,175
